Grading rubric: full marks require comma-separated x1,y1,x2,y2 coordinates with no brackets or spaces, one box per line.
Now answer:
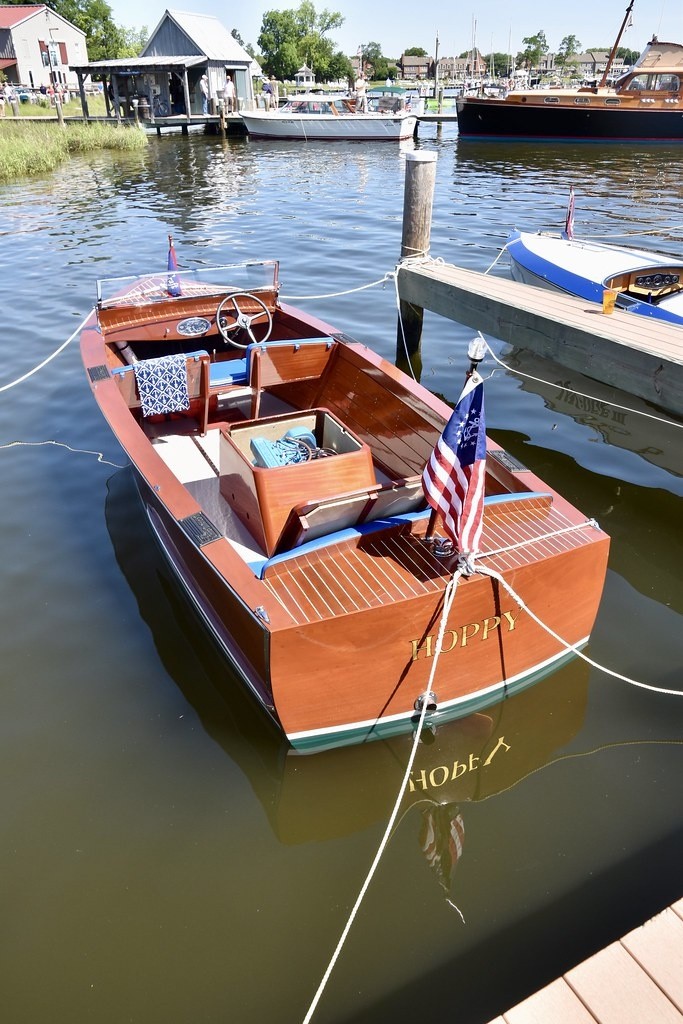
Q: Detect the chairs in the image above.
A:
110,336,339,437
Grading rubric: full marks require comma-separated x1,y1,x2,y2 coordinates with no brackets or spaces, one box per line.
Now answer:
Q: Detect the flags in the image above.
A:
167,246,182,294
421,374,486,558
625,15,633,32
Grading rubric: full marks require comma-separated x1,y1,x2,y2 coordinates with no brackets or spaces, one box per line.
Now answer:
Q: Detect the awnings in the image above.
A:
224,64,247,70
68,54,208,118
0,59,17,70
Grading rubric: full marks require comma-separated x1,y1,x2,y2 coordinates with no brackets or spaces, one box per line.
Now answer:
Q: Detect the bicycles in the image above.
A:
147,90,170,116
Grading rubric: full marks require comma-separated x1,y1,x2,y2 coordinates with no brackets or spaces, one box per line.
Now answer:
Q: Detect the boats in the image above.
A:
81,236,612,755
455,0,683,145
503,184,683,327
502,345,683,479
238,85,430,140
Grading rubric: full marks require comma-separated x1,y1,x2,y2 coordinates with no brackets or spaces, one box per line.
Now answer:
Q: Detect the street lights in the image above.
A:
44,39,65,124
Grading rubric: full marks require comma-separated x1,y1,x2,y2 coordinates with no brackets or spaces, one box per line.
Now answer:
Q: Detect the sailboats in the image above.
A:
403,16,620,118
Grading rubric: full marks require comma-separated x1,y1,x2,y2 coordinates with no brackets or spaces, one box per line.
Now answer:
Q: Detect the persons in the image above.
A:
47,81,64,109
107,78,116,116
353,72,370,114
199,75,209,116
98,81,110,95
223,75,235,115
3,81,11,106
262,75,279,112
40,83,46,95
